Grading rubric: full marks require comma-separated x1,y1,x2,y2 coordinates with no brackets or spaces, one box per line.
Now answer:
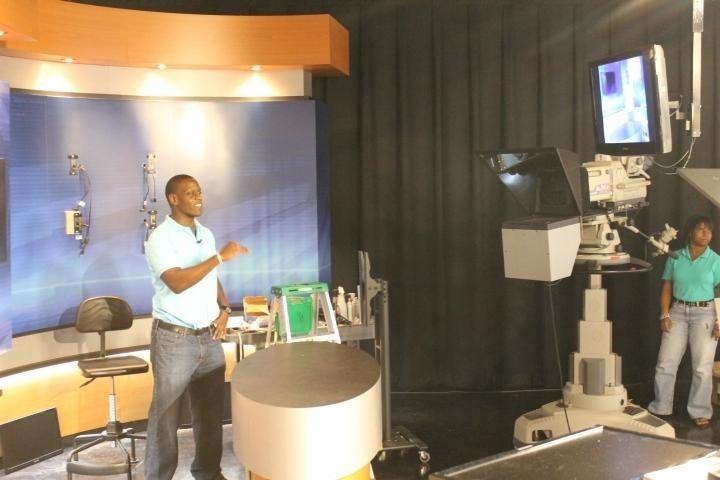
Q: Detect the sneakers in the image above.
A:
652,413,671,420
694,418,711,429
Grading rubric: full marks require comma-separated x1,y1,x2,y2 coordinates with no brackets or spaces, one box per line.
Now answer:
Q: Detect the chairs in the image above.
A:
63,292,151,479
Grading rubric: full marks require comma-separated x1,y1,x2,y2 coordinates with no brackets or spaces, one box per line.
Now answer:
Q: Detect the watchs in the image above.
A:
221,306,233,315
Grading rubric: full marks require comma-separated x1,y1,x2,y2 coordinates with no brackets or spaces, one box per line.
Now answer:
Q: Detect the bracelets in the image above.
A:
658,312,670,321
215,251,223,264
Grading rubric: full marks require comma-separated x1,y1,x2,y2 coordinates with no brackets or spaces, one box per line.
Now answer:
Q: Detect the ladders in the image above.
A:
265,282,341,349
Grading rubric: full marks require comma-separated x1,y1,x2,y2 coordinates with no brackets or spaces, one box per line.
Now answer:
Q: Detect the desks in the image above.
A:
220,315,380,370
229,340,383,480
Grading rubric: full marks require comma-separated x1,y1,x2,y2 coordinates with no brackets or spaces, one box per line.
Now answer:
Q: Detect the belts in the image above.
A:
672,297,714,307
151,318,215,335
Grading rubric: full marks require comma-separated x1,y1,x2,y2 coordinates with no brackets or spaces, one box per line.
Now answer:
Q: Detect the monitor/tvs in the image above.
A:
588,43,673,156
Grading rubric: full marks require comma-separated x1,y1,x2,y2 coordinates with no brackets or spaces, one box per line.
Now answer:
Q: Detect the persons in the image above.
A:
646,215,719,428
139,173,249,480
